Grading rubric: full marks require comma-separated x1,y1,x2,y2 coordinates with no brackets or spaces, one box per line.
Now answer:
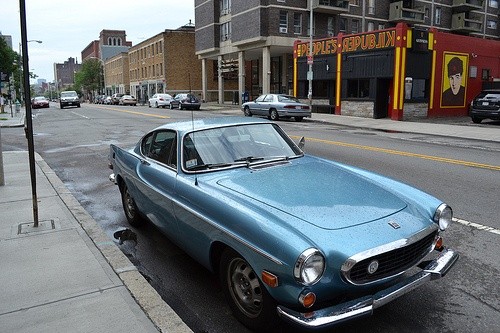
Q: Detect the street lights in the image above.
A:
89,56,107,96
19,39,43,107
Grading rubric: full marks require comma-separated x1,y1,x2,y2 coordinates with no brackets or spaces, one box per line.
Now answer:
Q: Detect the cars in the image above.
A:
45,97,58,102
31,96,50,109
119,95,137,106
107,115,460,331
148,93,174,109
469,89,500,126
241,93,311,122
94,95,111,105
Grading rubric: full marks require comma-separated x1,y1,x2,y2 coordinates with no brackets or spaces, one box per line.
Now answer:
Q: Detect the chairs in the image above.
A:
222,128,260,160
158,138,190,169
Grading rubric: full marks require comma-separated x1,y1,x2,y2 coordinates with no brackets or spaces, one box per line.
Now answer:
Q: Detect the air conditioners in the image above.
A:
280,27,287,33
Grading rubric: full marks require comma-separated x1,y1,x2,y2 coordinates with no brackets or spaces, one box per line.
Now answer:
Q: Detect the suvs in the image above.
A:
174,93,201,111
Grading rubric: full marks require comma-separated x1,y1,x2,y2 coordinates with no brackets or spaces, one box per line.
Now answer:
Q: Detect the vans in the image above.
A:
110,93,125,105
58,90,81,109
